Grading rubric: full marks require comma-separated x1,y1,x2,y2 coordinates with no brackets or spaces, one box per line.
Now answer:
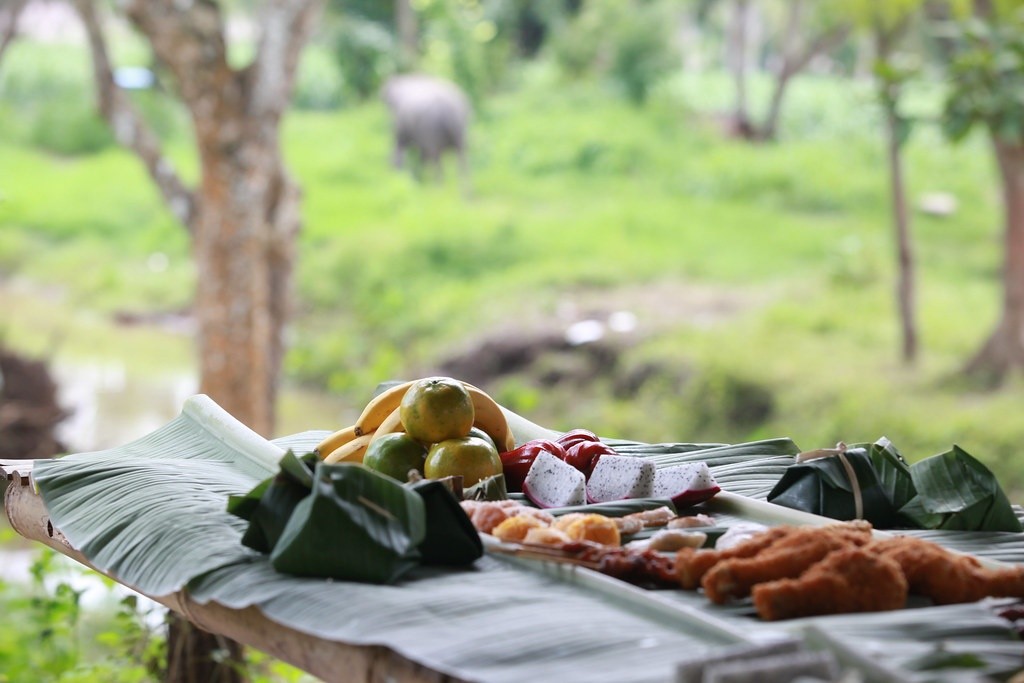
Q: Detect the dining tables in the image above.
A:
0,457,1024,683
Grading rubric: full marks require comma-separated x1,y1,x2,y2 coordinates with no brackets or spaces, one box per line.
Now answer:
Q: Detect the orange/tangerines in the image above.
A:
361,377,502,484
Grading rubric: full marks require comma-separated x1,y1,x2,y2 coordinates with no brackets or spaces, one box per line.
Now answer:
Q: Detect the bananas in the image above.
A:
306,379,515,464
